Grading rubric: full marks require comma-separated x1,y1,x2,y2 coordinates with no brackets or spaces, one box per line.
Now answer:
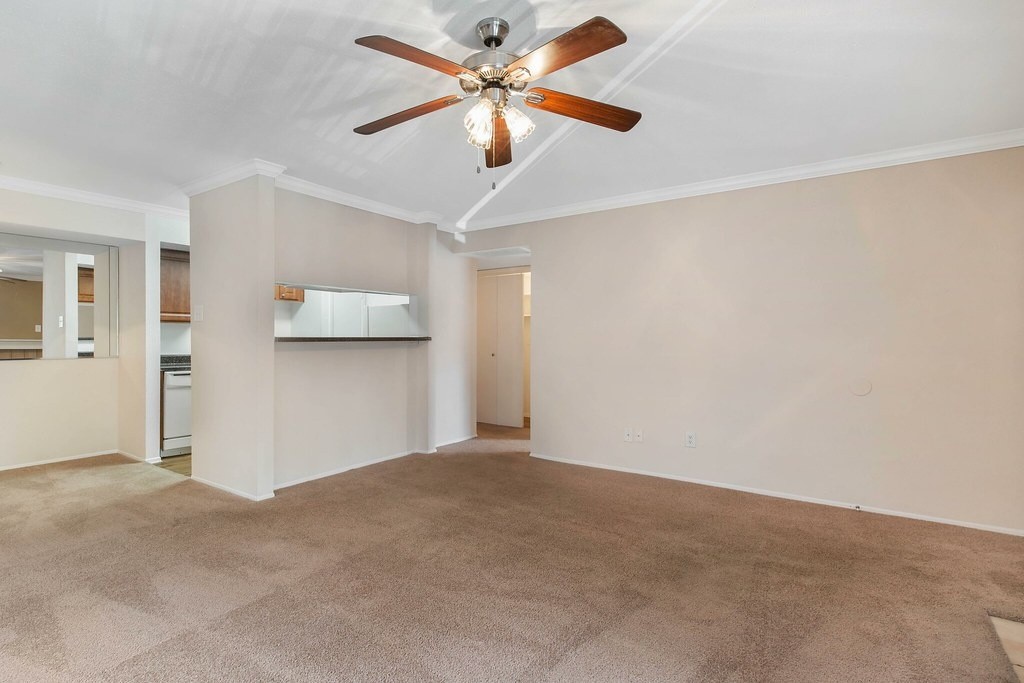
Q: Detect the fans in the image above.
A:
353,0,643,189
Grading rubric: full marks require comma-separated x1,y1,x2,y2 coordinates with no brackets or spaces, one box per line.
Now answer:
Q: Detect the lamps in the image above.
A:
463,97,536,149
